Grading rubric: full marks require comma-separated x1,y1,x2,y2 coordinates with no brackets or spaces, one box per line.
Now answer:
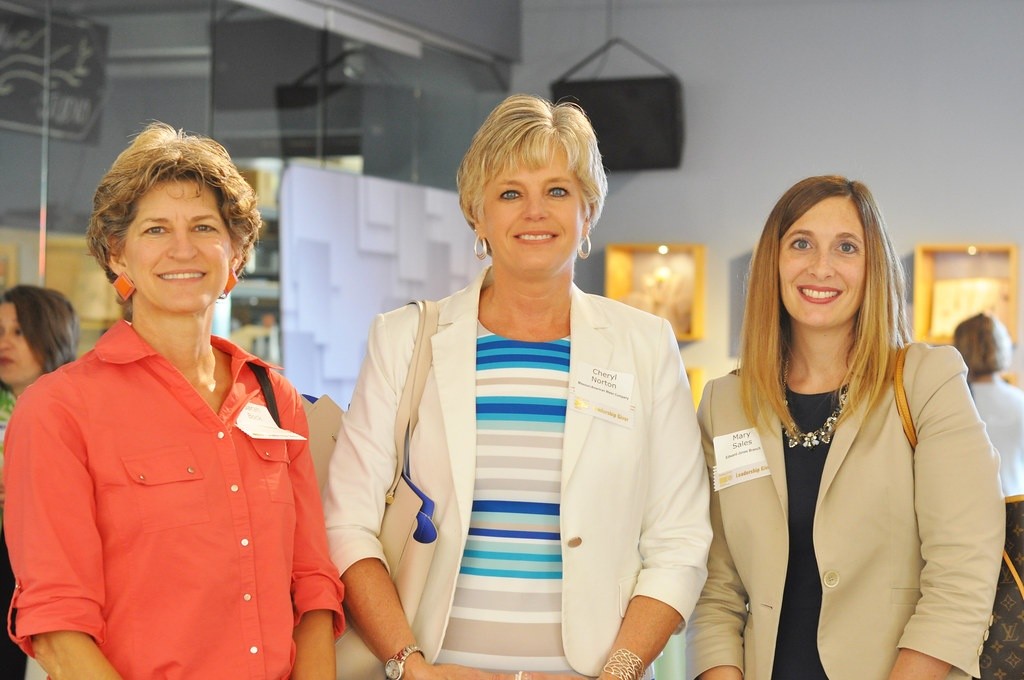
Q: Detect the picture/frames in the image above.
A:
912,242,1018,346
603,243,706,342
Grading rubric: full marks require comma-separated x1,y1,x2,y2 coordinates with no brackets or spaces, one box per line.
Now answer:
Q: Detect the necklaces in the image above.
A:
775,343,863,448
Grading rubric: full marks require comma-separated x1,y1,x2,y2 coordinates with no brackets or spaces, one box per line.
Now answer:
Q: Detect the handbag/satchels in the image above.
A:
894,341,1024,680
292,300,437,680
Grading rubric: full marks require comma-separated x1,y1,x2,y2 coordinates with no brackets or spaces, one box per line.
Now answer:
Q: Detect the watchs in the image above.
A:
385,643,426,680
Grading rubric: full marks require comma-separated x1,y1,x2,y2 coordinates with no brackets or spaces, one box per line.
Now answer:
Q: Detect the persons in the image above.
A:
3,121,344,680
0,284,81,680
320,95,713,678
954,310,1024,493
696,176,1007,678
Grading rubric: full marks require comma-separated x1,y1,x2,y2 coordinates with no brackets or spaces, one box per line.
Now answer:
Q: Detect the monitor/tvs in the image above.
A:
549,76,683,173
